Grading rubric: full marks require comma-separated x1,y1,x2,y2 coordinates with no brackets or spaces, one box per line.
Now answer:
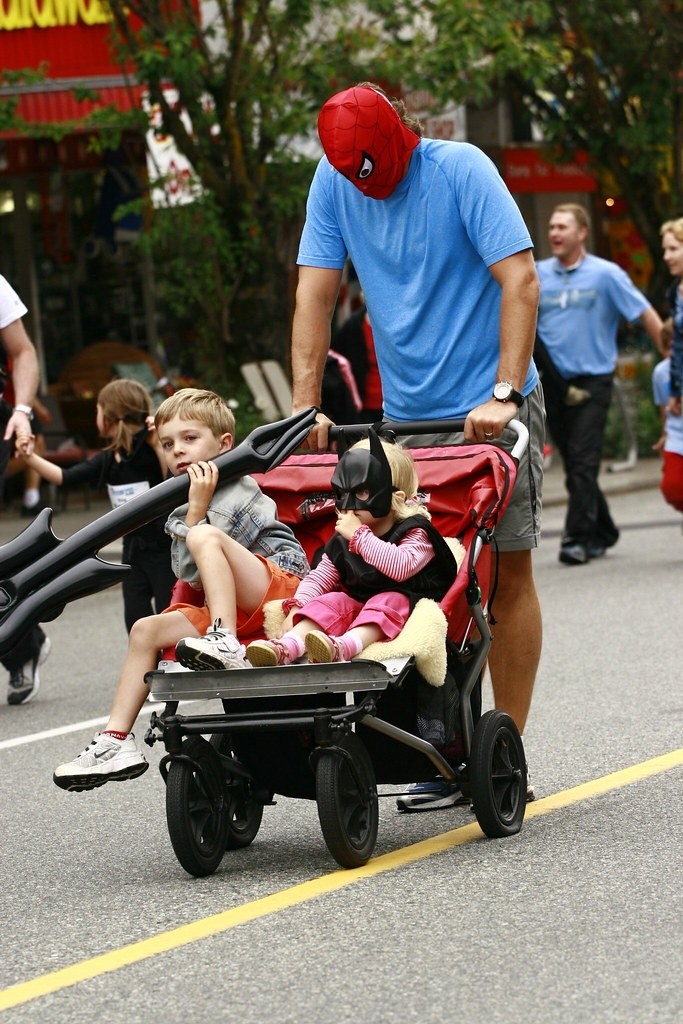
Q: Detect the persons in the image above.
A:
531,203,664,564
15,379,177,703
325,290,385,453
54,387,311,791
292,81,547,811
651,217,683,514
246,430,457,669
0,275,46,519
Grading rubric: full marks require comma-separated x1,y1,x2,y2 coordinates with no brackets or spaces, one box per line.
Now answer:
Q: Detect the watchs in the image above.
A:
16,405,34,420
492,382,525,408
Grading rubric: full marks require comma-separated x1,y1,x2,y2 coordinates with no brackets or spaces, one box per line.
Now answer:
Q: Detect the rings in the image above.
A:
485,433,493,437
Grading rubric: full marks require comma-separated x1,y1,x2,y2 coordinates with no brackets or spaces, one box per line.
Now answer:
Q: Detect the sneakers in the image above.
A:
396,761,470,812
559,531,588,564
53,732,149,793
7,636,51,705
175,617,253,672
526,772,535,802
587,536,607,556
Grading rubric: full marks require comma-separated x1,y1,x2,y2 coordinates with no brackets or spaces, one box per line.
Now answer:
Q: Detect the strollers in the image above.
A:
137,414,537,877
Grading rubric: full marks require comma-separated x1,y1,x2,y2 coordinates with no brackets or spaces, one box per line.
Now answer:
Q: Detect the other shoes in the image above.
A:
246,638,290,668
21,499,59,518
305,630,345,664
